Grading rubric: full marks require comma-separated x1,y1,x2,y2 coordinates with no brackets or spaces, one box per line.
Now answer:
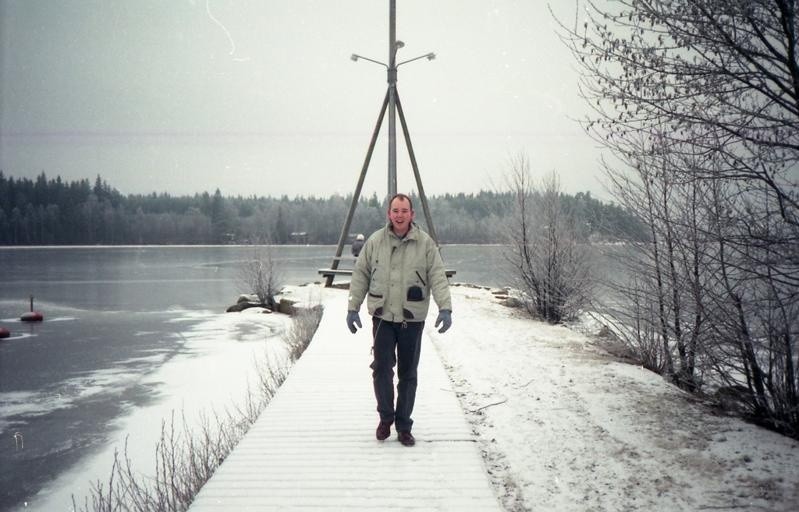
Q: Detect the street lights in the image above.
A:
350,40,436,224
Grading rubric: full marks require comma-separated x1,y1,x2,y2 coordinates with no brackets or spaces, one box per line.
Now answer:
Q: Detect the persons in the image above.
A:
346,194,451,447
350,231,365,260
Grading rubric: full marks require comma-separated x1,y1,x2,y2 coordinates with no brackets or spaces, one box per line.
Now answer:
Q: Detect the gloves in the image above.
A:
346,310,362,333
435,310,452,334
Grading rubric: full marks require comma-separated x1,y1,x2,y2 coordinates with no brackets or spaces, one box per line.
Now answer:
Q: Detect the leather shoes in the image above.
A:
376,420,393,440
397,430,415,446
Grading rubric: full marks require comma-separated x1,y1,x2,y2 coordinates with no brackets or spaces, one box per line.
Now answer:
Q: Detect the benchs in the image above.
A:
318,268,456,287
